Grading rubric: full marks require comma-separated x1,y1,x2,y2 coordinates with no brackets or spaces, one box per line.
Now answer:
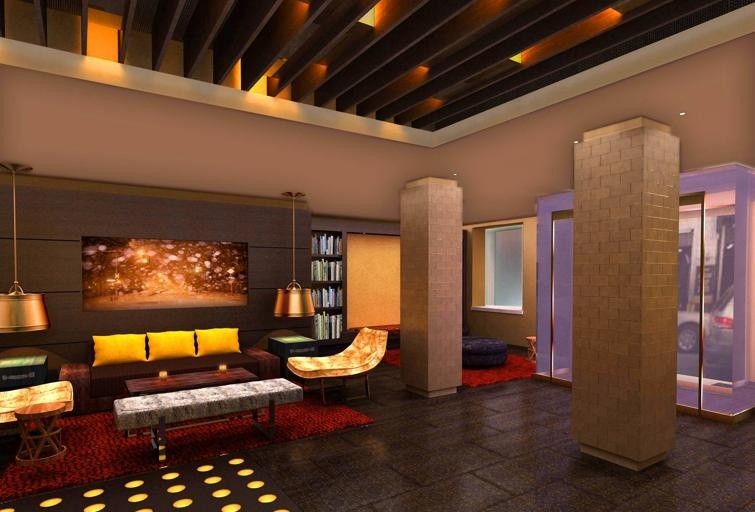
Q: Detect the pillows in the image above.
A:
92,327,243,368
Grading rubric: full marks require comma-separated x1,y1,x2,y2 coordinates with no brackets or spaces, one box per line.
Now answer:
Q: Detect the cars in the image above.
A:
678,308,714,356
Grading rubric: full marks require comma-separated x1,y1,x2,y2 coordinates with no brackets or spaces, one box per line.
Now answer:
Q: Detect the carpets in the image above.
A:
1,390,374,503
381,347,537,389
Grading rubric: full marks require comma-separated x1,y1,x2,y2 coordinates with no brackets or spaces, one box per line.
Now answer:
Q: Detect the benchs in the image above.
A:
113,378,304,462
350,324,401,348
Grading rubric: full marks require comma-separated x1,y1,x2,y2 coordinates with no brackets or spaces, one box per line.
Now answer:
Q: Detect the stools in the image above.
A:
462,336,509,369
14,401,68,466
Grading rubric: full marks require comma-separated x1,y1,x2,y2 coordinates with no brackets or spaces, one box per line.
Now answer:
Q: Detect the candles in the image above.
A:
219,365,226,371
159,371,168,378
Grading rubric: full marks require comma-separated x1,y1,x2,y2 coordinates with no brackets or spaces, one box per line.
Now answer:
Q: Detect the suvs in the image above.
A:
705,281,737,369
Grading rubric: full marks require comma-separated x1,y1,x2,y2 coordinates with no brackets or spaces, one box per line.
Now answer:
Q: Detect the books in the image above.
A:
310,231,344,340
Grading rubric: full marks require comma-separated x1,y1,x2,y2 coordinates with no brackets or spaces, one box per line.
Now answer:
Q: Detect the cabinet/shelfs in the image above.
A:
310,224,348,344
268,334,318,382
0,354,49,392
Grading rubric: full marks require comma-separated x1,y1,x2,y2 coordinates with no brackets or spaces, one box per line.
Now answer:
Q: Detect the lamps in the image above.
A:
0,161,51,334
273,192,315,318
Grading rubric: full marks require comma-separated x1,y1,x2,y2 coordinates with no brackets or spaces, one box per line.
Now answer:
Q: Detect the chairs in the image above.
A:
0,380,74,427
287,328,388,404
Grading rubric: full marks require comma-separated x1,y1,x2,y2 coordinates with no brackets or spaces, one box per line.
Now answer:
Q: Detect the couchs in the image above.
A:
58,336,281,412
